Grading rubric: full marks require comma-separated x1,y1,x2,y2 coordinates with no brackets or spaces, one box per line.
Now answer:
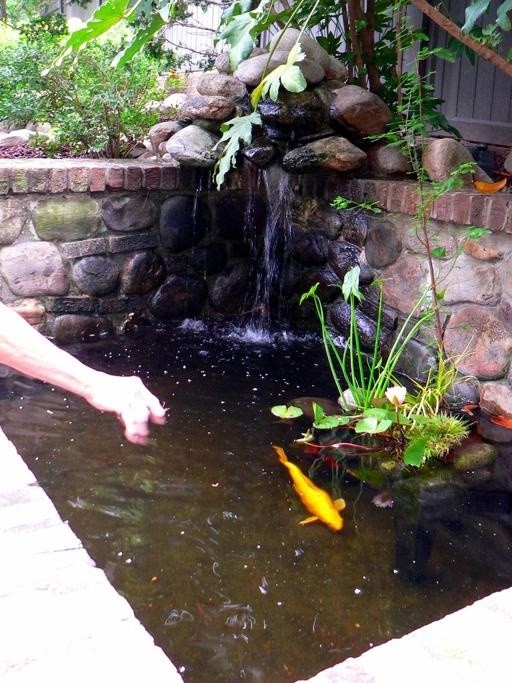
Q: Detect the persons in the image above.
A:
1,301,169,446
